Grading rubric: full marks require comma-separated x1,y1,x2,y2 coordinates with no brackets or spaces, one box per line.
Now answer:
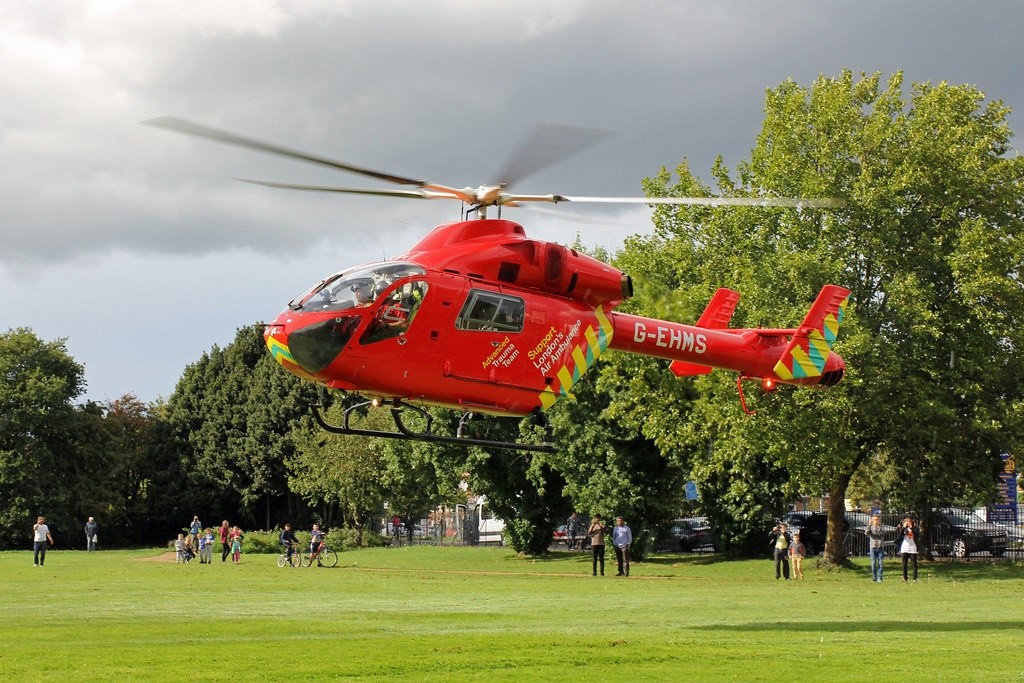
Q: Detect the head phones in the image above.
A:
368,282,376,300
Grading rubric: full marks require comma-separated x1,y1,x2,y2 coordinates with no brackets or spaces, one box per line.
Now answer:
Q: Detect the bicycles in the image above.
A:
277,542,302,568
302,540,339,568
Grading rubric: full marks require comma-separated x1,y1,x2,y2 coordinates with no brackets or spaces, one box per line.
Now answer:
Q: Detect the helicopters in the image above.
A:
142,116,854,454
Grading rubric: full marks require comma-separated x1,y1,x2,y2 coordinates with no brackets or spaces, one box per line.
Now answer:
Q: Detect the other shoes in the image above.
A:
40,564,43,567
34,564,38,567
785,577,792,581
794,577,798,580
871,580,882,583
800,575,804,580
913,580,917,583
615,571,624,576
902,579,907,583
776,577,781,580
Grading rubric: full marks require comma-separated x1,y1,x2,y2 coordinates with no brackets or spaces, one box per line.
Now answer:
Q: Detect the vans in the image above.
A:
456,492,510,544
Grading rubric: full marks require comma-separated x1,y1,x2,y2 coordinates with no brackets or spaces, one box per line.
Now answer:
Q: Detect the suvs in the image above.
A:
801,510,910,558
909,507,1008,559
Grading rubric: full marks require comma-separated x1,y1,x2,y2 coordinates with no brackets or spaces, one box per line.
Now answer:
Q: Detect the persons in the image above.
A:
865,515,886,584
788,534,806,581
769,522,792,582
588,514,606,577
612,516,632,576
566,511,586,550
404,515,415,542
392,514,400,541
308,523,325,567
85,517,97,552
281,523,301,567
33,515,54,568
334,280,408,341
893,516,921,582
175,515,244,564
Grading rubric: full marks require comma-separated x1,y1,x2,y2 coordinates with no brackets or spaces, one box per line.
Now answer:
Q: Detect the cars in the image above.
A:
387,523,424,536
553,525,591,549
651,519,712,551
782,511,811,535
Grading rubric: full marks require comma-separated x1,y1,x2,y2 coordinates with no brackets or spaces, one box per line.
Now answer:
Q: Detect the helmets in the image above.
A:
376,280,397,298
351,279,375,299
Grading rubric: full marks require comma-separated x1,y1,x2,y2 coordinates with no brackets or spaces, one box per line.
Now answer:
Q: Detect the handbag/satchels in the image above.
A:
92,533,97,543
